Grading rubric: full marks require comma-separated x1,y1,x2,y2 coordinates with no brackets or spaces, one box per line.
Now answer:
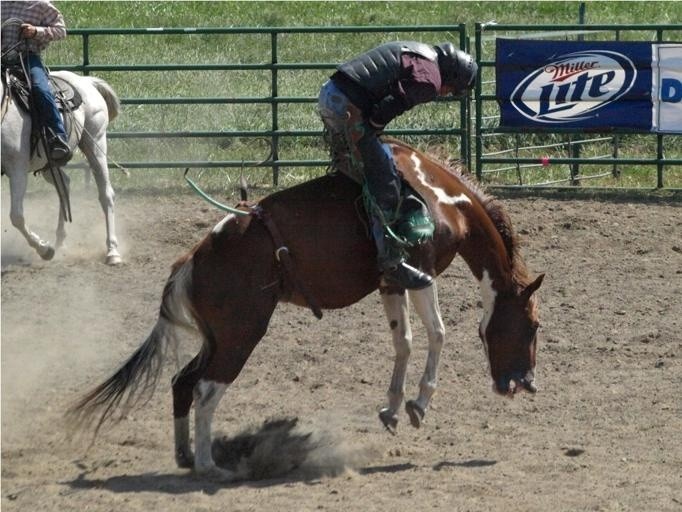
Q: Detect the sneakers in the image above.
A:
51,145,67,160
380,262,433,289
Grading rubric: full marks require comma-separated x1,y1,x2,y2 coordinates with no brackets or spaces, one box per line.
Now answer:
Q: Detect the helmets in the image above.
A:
433,43,479,97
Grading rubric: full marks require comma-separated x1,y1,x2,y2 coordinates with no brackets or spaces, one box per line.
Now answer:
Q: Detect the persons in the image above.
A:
319,39,480,295
2,1,73,167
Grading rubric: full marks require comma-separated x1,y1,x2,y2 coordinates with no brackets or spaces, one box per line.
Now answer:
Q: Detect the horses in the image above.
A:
1,65,123,266
65,132,548,479
